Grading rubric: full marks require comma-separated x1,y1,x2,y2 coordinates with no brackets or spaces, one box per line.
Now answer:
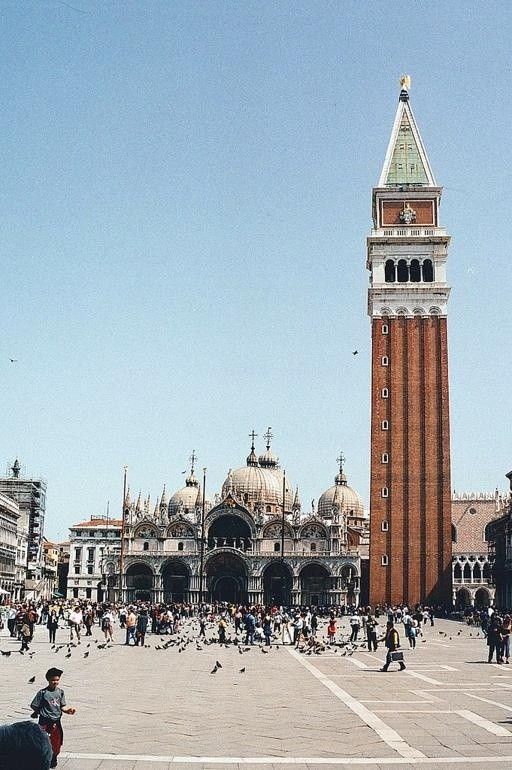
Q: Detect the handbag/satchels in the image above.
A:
389,650,405,663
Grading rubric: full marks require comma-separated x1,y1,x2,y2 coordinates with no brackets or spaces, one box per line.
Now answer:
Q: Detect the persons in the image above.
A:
29,667,77,768
480,608,490,639
485,619,504,665
1,593,436,654
499,614,511,664
375,620,406,672
0,718,54,770
437,602,512,630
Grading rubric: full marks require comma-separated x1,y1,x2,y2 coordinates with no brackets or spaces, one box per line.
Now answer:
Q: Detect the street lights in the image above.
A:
198,465,208,605
118,463,130,605
280,468,288,607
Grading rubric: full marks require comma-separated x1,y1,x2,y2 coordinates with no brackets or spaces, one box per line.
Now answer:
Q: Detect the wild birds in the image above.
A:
239,668,245,673
9,358,18,362
352,350,358,355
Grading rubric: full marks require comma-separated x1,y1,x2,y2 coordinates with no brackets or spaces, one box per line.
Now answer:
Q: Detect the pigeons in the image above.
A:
29,676,35,683
422,640,426,643
144,616,384,657
211,666,217,674
439,629,480,640
216,661,222,668
51,638,113,658
0,650,35,659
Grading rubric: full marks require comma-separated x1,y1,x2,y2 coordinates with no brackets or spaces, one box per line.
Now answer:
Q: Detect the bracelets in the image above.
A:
395,647,397,648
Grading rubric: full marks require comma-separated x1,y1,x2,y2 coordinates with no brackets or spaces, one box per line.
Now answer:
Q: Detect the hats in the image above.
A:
45,667,64,679
